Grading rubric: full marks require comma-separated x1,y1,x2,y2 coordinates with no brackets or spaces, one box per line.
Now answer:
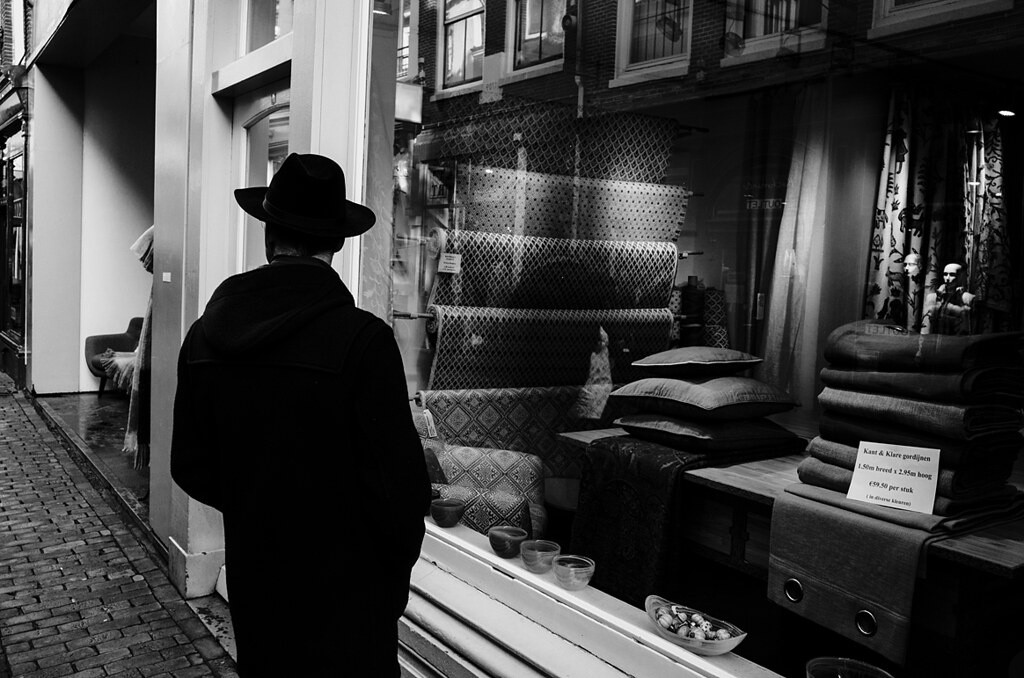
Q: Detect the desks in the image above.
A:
558,411,1023,670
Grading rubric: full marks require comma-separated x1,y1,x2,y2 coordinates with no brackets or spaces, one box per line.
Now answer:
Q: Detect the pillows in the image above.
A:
614,346,810,452
423,438,548,541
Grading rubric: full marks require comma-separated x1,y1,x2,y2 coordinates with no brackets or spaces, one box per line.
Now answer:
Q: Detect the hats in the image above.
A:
234,153,376,239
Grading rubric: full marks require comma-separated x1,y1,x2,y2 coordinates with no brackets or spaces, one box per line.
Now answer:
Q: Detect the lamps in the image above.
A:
656,0,801,68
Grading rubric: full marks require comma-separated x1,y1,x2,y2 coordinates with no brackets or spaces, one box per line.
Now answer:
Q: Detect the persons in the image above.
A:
169,151,431,678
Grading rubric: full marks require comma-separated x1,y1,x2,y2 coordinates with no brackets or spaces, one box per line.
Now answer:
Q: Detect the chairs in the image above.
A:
84,316,146,401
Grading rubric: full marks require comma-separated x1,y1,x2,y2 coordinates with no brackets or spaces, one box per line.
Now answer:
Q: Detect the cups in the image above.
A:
806,657,893,678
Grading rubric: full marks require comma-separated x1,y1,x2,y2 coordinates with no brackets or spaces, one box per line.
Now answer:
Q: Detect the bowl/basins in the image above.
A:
488,526,528,559
520,539,560,574
431,499,466,528
552,554,595,591
426,490,441,515
